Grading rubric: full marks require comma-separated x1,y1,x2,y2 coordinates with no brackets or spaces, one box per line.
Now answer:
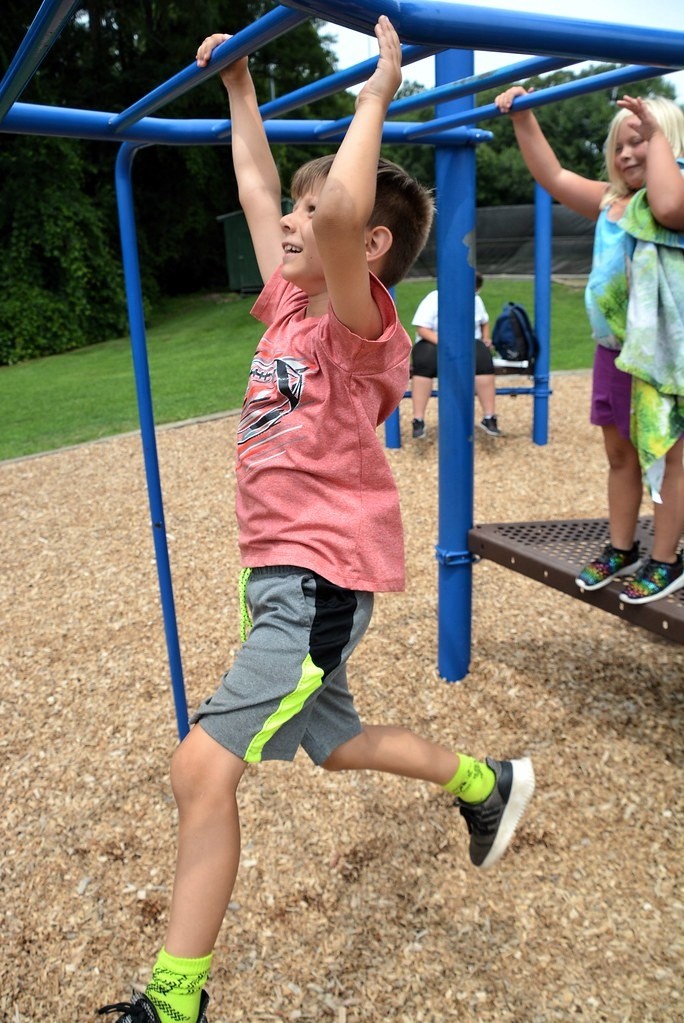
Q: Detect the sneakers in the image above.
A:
459,759,536,866
620,547,684,604
410,418,427,440
574,540,643,590
479,414,503,435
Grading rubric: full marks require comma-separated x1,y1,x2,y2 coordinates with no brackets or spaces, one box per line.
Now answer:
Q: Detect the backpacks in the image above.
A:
489,299,538,372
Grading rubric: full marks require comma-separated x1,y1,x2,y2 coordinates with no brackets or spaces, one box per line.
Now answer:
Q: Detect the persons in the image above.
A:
99,16,533,1023
408,266,504,442
493,78,684,609
